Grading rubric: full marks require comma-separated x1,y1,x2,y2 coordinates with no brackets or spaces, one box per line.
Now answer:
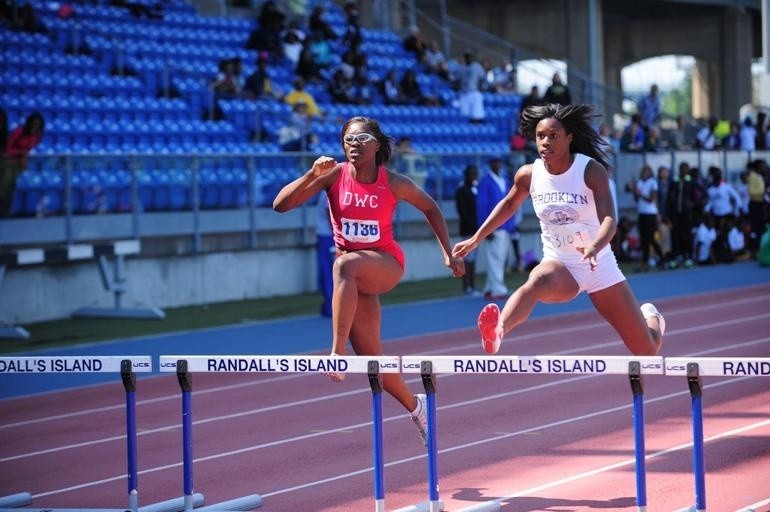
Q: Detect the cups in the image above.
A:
343,132,378,145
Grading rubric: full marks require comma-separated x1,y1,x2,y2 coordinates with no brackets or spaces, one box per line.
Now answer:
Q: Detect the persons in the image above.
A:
210,51,325,151
387,136,417,176
451,103,667,354
453,156,522,300
315,189,335,318
124,1,165,21
244,1,518,106
55,1,76,21
0,111,44,217
1,1,49,38
597,83,769,273
272,115,464,447
447,47,487,124
512,70,572,156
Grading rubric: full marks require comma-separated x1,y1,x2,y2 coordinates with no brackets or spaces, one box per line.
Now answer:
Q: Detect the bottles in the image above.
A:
258,51,270,62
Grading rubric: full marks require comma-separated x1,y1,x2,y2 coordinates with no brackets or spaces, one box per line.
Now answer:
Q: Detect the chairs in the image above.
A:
684,259,694,268
649,258,658,271
410,393,428,447
476,302,505,355
0,0,526,216
313,352,347,383
668,260,678,270
633,264,650,273
639,302,666,337
